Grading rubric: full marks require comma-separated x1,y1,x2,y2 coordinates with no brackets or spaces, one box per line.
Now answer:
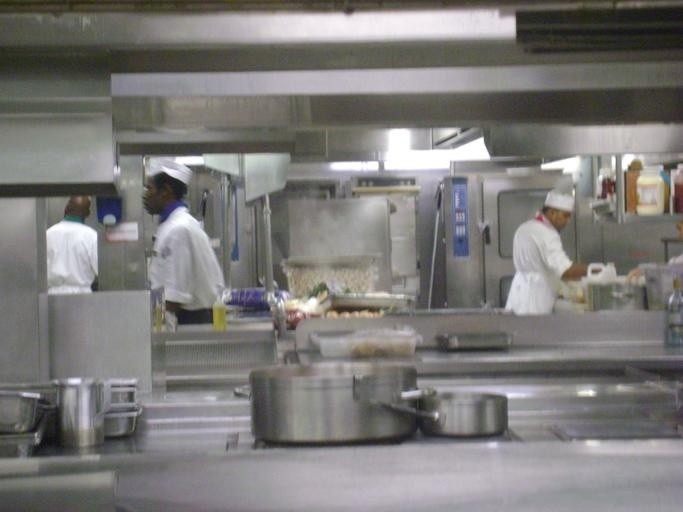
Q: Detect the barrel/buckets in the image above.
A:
636,169,664,217
588,261,616,280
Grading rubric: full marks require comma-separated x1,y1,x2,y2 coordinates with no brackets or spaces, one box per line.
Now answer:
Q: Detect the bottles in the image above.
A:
212,297,227,332
668,278,682,346
673,163,683,216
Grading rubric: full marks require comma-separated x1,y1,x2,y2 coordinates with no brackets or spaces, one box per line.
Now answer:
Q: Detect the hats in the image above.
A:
149,158,192,186
546,193,574,213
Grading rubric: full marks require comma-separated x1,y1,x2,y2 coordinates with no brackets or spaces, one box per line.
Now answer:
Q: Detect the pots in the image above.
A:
645,267,682,310
249,364,508,446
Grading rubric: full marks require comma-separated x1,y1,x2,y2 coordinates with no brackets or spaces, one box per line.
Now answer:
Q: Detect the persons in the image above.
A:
138,156,226,325
41,193,99,295
505,191,602,317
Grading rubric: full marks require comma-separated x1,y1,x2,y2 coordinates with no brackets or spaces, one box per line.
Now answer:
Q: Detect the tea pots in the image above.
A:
53,377,112,449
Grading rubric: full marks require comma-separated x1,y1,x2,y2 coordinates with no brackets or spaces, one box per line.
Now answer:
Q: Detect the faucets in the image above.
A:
260,190,277,303
